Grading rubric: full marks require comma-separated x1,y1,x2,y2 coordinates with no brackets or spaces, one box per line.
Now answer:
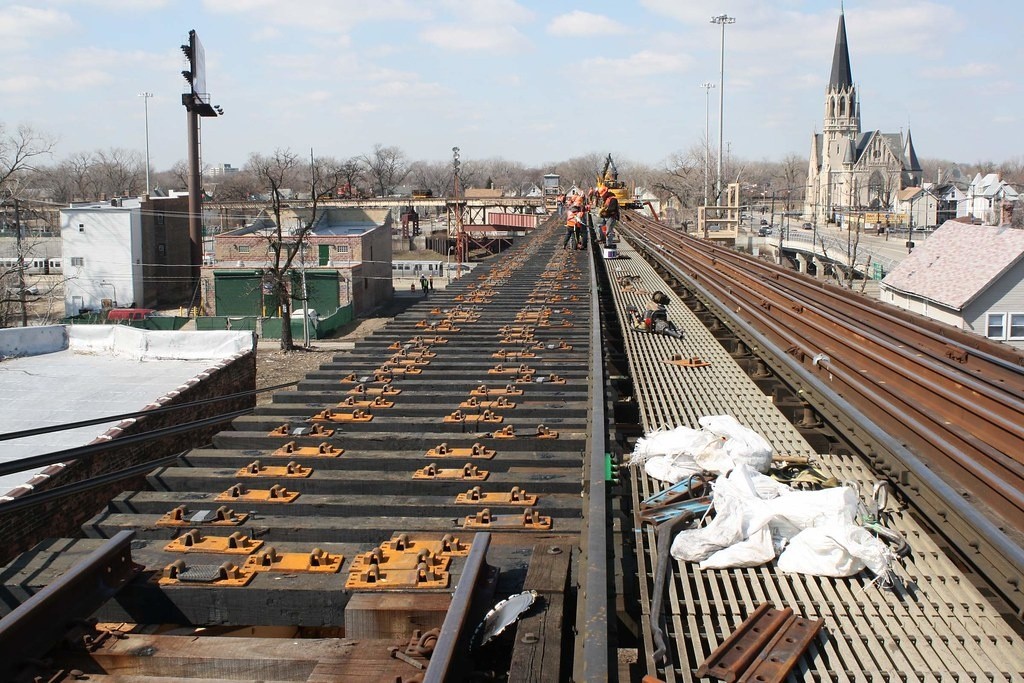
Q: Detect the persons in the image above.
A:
558,187,620,249
420,274,434,293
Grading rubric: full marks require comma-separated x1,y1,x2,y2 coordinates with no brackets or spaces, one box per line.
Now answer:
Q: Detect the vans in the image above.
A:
108,308,162,321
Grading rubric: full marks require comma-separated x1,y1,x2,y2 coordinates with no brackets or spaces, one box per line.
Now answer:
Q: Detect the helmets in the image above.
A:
598,186,608,197
575,196,584,204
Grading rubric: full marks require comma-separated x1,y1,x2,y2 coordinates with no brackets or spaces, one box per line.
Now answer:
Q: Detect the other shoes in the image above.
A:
595,236,606,243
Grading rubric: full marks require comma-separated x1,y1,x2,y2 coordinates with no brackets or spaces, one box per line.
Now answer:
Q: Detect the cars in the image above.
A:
6,282,39,295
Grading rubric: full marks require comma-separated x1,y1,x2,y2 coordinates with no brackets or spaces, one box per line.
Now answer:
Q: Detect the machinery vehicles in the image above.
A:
588,153,645,208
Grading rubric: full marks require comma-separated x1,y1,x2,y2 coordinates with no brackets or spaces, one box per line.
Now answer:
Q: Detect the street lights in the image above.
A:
701,82,715,205
138,93,154,194
847,176,881,265
813,182,843,245
448,246,454,284
771,185,812,241
711,14,736,218
452,147,460,282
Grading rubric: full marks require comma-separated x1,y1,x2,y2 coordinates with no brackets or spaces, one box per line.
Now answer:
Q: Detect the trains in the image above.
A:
0,257,472,277
